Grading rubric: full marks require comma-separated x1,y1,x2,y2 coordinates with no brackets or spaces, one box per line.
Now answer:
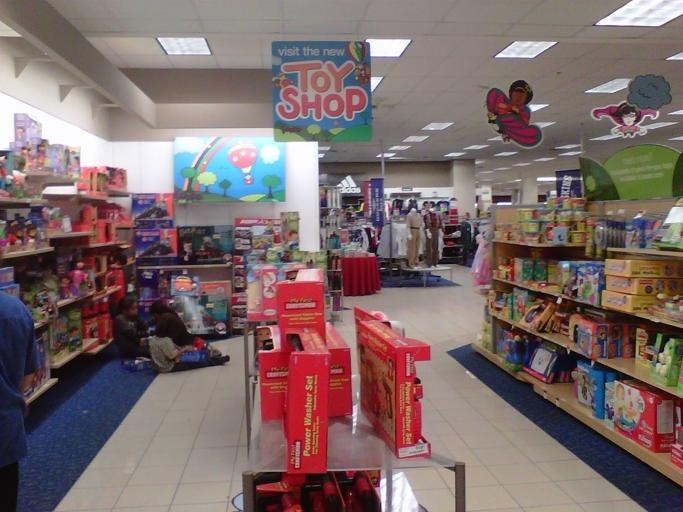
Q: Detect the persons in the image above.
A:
423,208,439,268
407,208,421,268
148,313,230,373
137,300,194,346
112,296,151,357
0,293,41,511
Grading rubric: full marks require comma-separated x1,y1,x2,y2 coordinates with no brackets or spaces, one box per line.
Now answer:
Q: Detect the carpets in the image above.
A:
15,341,161,512
446,343,683,512
332,268,463,291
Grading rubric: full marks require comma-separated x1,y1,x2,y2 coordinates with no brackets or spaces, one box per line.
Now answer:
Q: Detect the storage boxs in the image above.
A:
245,268,431,475
132,191,234,336
612,379,676,453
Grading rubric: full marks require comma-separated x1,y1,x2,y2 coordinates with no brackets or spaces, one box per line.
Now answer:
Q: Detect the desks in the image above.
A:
331,256,381,295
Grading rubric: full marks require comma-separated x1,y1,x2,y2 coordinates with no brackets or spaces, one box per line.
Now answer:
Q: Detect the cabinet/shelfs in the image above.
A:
471,199,683,486
243,316,465,511
0,174,132,419
441,224,464,264
320,185,343,250
